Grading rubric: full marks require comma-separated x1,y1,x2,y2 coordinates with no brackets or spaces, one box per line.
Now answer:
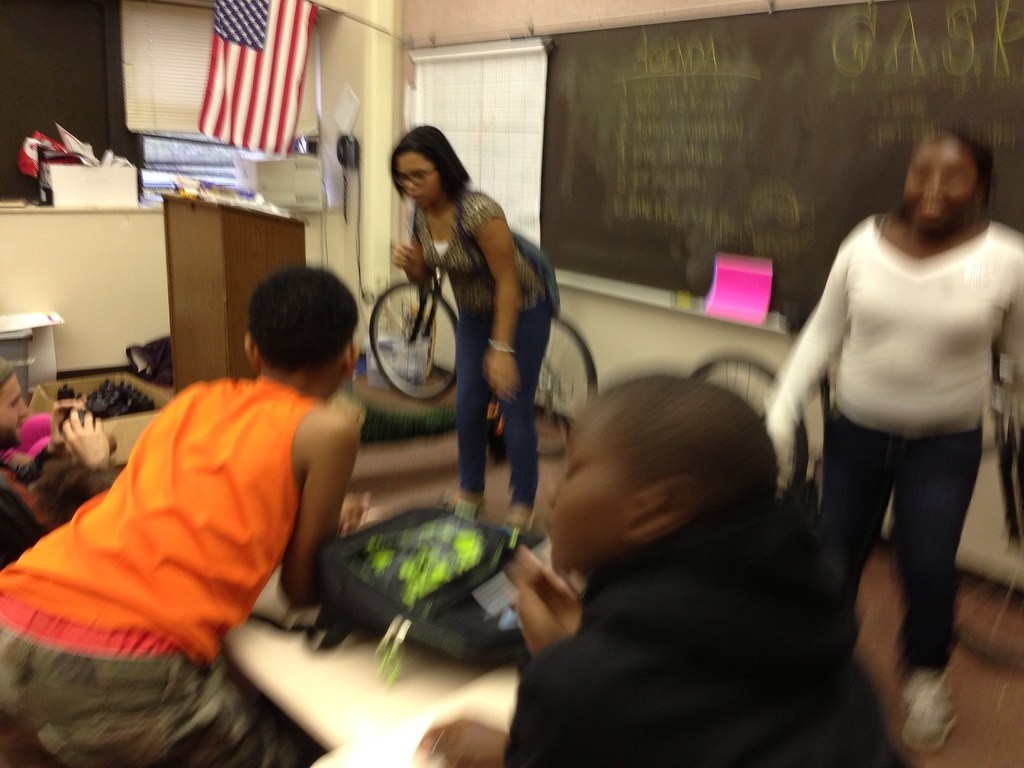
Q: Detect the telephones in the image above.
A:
336,135,361,171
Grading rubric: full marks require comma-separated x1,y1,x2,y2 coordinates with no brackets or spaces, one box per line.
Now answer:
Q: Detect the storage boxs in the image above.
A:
28,372,174,468
38,161,138,209
243,151,323,209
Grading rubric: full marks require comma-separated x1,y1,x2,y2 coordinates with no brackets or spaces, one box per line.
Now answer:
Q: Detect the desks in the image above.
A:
223,562,523,767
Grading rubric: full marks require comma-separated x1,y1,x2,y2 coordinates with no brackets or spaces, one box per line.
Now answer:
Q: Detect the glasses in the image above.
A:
397,170,436,186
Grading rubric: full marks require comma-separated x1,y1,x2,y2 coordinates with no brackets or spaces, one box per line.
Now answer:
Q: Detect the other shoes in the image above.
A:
902,672,956,750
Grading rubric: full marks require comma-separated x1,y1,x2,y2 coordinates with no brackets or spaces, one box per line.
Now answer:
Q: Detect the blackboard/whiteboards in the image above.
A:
541,3,1024,349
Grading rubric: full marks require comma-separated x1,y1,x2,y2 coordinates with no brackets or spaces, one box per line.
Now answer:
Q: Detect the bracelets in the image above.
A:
489,340,516,354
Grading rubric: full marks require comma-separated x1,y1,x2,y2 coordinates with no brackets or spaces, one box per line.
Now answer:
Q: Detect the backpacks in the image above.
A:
512,230,559,318
289,506,586,672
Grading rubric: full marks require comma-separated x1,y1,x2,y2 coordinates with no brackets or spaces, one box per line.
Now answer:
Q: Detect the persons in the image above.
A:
764,128,1024,751
198,0,316,156
390,126,558,536
419,374,909,768
0,254,360,768
